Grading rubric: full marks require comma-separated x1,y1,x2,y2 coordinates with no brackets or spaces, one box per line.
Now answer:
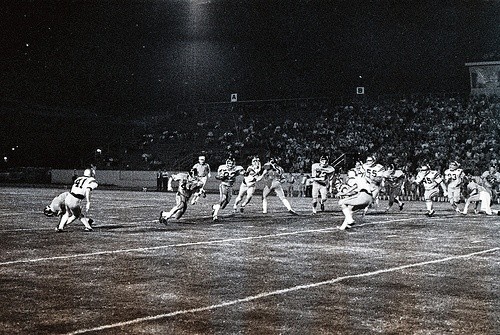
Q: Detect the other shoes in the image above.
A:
55,219,94,233
159,201,500,230
203,190,208,198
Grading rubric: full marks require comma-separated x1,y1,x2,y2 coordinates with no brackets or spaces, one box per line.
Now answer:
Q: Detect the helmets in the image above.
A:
349,156,496,188
320,156,329,166
44,206,55,217
84,168,95,177
251,157,260,166
226,157,235,168
269,157,278,166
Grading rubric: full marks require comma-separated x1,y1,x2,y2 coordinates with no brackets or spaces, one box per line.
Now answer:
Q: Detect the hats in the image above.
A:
199,156,205,160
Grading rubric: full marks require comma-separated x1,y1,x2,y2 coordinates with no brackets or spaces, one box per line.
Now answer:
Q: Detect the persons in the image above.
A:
460,175,500,216
337,155,385,228
286,160,312,197
57,168,98,229
415,161,447,217
311,156,336,214
43,192,94,231
404,163,425,201
481,166,500,207
329,172,345,198
162,168,169,192
159,167,203,226
463,172,483,188
72,169,79,179
122,90,500,162
234,157,267,214
262,157,297,215
156,168,162,192
190,155,211,205
460,162,500,176
91,164,97,177
383,163,406,212
444,161,466,212
212,158,245,221
